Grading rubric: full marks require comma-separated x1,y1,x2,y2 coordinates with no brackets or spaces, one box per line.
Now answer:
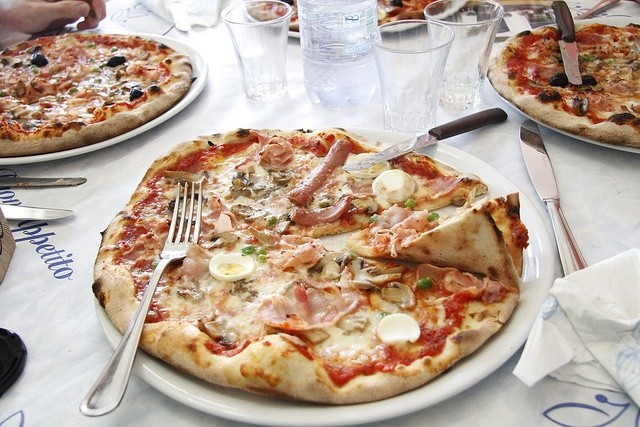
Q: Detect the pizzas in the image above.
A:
485,16,640,150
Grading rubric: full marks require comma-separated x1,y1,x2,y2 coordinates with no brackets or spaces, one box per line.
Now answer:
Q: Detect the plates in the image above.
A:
485,66,640,154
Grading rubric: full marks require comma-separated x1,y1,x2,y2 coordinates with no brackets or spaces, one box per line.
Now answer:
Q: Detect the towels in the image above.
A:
513,247,639,404
138,0,220,31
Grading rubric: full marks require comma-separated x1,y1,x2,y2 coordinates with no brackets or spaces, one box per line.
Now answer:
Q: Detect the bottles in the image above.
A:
296,0,383,115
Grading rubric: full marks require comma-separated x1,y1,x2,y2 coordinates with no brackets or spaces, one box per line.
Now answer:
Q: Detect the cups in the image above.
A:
423,0,505,113
368,17,456,141
219,0,293,103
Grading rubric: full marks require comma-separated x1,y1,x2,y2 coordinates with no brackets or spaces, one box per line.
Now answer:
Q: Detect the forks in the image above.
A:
79,179,206,419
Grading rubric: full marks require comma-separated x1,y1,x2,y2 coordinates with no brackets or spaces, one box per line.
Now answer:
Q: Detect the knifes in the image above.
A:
518,118,589,278
550,0,583,86
0,175,89,188
165,0,192,32
342,106,508,173
0,204,74,221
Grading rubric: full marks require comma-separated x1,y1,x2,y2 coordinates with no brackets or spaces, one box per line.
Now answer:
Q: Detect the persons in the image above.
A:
0,0,106,42
